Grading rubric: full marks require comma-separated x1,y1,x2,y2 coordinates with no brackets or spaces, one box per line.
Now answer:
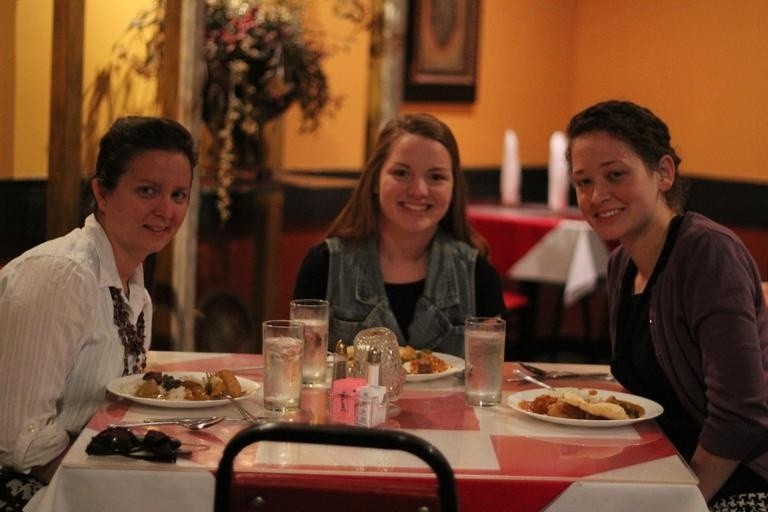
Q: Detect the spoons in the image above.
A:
518,363,611,380
106,413,229,434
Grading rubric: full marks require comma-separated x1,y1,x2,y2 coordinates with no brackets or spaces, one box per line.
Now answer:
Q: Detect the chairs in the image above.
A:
214,422,457,512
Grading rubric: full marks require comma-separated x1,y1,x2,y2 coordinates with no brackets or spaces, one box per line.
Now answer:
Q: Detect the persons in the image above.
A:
291,111,509,360
565,101,768,511
1,115,197,512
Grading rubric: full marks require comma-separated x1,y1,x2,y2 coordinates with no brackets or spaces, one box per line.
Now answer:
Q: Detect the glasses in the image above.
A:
85,427,182,465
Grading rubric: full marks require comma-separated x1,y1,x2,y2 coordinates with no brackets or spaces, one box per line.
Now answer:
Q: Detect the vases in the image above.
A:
202,84,299,193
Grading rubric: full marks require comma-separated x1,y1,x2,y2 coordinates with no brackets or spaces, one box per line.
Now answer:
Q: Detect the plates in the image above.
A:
507,387,664,428
398,346,465,381
397,375,467,402
106,371,260,409
494,410,662,448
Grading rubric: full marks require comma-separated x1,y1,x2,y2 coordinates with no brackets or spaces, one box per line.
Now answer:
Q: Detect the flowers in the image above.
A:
82,0,329,221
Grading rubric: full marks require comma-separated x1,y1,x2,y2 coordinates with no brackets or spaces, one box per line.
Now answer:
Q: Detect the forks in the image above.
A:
203,368,256,424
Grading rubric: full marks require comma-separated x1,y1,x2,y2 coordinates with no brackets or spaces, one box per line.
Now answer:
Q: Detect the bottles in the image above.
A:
354,347,387,427
330,338,348,381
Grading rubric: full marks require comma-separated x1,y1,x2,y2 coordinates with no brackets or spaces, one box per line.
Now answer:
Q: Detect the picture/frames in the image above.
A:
406,0,479,100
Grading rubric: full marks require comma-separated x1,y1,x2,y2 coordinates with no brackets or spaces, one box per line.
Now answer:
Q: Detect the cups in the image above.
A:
459,401,502,468
288,298,330,386
463,314,507,405
260,319,304,411
259,413,300,468
300,386,335,468
